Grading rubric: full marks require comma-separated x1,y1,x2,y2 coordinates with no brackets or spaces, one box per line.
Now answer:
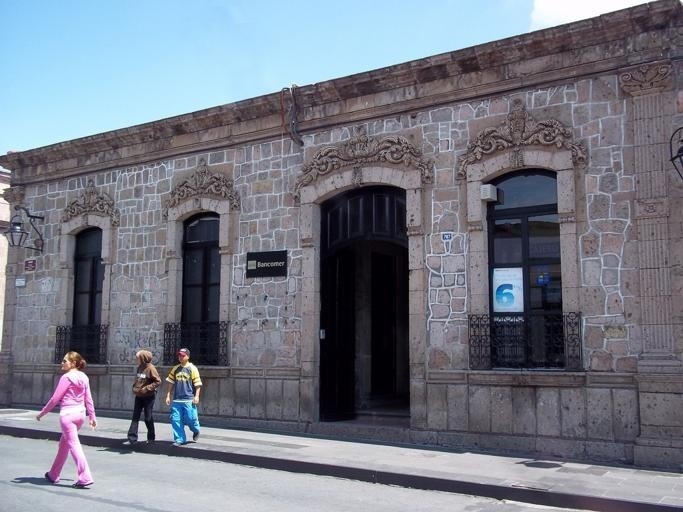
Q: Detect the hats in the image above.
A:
174,348,191,358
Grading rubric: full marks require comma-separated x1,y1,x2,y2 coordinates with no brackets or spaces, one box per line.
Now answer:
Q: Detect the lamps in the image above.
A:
667,126,683,184
3,204,44,247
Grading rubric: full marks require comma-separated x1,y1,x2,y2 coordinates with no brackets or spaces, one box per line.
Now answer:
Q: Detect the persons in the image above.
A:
122,350,161,446
165,348,203,447
35,351,98,488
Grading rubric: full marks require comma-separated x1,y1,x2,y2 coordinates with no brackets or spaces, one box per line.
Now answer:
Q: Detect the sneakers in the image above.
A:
120,440,155,449
45,471,56,485
172,431,200,446
71,481,94,488
